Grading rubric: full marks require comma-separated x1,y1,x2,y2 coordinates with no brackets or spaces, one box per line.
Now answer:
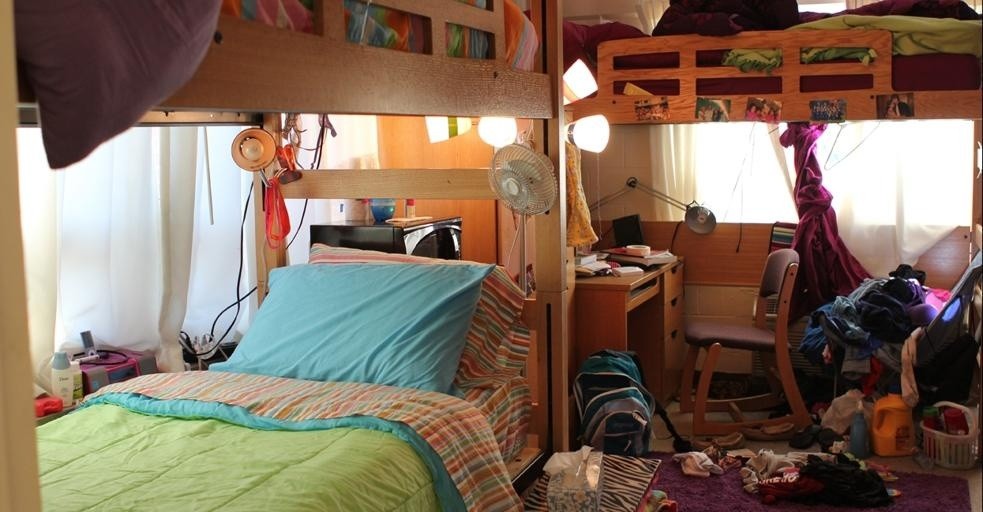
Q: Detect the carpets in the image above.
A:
636,452,971,512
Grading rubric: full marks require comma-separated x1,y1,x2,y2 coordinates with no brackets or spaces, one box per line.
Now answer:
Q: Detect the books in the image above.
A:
574,247,678,267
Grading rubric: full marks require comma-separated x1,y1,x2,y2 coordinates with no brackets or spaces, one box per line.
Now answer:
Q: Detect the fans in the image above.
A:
488,137,558,292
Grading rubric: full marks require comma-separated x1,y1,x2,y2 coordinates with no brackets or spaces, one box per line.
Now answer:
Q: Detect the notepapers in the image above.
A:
612,266,644,277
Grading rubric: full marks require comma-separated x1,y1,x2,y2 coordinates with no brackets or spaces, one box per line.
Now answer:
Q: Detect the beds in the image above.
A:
575,31,982,126
0,1,567,510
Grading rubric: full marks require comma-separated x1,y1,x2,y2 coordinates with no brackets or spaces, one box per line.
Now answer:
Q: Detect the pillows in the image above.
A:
207,259,500,393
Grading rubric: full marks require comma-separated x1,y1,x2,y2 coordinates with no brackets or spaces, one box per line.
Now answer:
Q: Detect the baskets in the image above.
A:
918,400,978,473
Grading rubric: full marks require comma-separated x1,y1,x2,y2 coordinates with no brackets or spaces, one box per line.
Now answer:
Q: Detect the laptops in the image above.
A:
612,214,645,247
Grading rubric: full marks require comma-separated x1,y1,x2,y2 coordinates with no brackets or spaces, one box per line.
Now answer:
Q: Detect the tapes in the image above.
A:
626,245,651,256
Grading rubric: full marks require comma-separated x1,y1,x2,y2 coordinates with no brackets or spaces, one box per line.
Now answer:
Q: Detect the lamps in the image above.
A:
562,58,598,107
232,128,276,188
567,114,610,153
586,176,716,235
421,116,472,143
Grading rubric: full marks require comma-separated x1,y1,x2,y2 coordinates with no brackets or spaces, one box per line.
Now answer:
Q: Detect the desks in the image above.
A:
575,257,684,402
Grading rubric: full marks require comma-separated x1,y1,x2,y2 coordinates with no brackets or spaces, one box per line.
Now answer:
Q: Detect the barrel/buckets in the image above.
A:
871,385,916,456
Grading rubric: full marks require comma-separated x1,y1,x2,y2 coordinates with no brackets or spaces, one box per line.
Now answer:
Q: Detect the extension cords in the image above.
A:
196,335,219,359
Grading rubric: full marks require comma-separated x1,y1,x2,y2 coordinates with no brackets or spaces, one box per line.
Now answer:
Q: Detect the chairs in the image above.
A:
820,249,983,400
680,249,813,436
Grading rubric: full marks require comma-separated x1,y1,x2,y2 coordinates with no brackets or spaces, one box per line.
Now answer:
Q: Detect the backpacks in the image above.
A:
573,347,692,455
